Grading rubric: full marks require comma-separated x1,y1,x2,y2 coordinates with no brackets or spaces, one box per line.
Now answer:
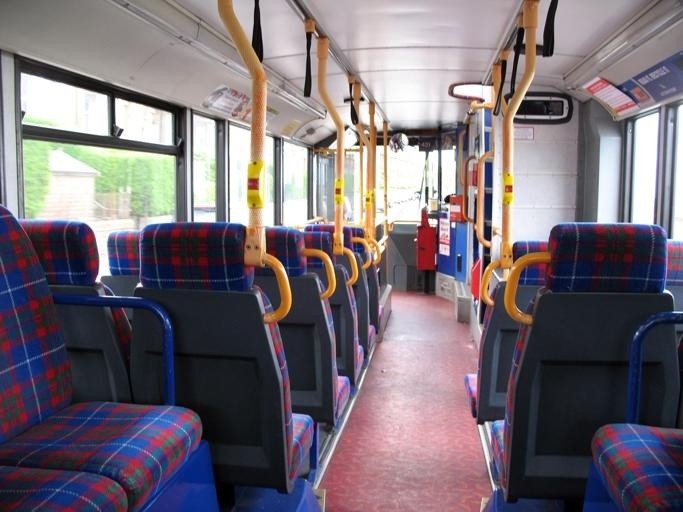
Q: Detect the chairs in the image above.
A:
0,204,382,512
466,223,683,511
